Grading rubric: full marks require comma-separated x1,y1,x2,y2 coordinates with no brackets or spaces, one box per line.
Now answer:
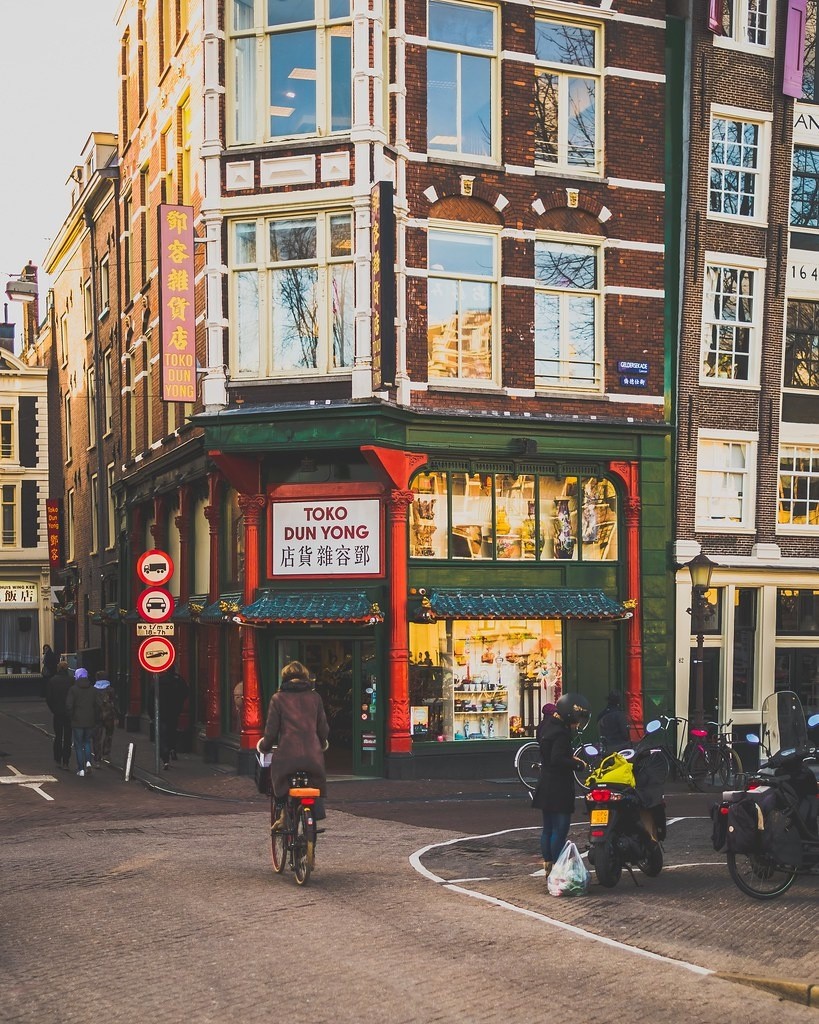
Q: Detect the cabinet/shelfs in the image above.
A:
453,690,510,741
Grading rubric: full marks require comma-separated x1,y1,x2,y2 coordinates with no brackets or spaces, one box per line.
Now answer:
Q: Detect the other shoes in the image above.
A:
76,770,85,776
171,750,177,760
271,815,285,831
162,763,169,770
85,761,92,767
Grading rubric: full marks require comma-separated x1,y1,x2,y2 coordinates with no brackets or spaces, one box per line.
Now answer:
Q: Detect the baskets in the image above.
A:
254,753,270,793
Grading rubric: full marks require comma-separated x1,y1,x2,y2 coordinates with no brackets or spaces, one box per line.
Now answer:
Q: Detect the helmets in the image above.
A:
557,693,591,724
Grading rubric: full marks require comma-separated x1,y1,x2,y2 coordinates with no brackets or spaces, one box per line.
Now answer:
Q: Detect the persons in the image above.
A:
256,661,330,871
531,694,591,881
536,703,556,745
597,690,631,757
66,668,97,777
45,662,74,770
92,670,120,769
42,644,57,698
148,664,188,769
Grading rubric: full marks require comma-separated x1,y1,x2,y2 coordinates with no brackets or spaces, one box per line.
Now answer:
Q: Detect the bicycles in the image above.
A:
725,775,819,901
631,715,745,793
513,712,602,791
254,745,326,886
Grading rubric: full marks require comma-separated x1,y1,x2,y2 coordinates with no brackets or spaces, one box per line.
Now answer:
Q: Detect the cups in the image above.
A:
463,683,482,691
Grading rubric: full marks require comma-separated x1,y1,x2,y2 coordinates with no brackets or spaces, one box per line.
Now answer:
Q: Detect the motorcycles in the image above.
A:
585,719,666,889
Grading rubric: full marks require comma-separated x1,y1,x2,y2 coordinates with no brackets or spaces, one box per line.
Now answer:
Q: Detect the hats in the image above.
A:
58,661,68,670
542,703,555,715
75,668,88,679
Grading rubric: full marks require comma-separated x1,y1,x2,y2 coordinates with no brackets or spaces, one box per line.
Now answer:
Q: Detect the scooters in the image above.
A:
732,689,819,841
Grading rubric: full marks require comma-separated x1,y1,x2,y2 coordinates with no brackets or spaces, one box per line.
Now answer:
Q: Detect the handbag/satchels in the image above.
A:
585,752,636,788
547,840,591,896
